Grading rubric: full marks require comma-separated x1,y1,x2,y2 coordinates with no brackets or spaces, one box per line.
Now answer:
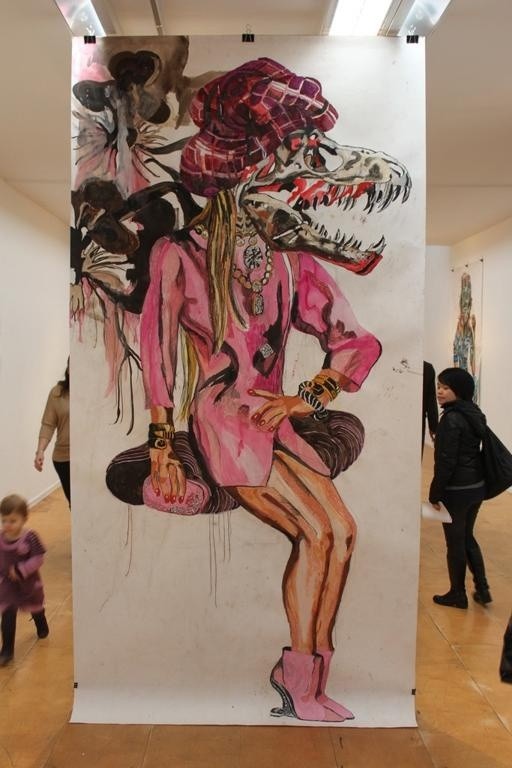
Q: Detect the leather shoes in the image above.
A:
473,590,492,604
433,592,468,609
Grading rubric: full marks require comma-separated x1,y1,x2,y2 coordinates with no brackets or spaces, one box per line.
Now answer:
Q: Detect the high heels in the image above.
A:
316,649,355,720
270,646,345,722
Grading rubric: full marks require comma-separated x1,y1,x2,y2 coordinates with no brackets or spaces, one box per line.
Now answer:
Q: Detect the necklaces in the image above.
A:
197,221,275,318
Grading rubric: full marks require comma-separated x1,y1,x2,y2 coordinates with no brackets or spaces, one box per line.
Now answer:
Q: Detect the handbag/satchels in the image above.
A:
480,423,512,501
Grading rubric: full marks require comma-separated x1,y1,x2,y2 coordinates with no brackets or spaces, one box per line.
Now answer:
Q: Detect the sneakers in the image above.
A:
0,649,14,666
35,618,49,638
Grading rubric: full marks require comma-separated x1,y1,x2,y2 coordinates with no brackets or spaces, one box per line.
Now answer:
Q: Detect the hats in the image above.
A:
178,57,339,198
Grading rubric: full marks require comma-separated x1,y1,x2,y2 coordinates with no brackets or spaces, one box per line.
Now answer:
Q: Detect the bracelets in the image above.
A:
35,448,44,454
310,370,341,401
149,419,178,449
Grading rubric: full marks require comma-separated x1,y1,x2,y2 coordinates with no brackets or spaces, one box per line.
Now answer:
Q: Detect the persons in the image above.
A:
1,493,51,666
134,54,382,724
428,366,492,610
34,352,70,510
422,362,441,461
